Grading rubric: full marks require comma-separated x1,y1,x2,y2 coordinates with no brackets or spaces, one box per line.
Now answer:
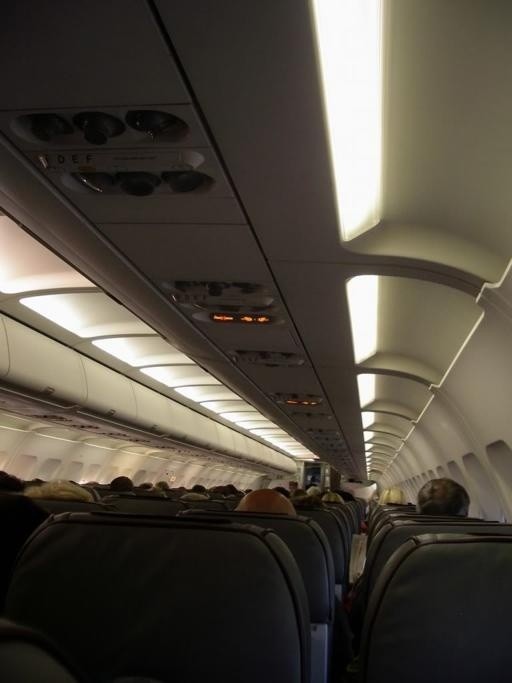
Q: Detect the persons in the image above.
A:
0,469,470,518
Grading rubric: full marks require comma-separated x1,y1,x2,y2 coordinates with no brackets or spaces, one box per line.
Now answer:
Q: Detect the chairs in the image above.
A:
3,491,54,620
1,623,82,681
13,512,330,681
177,509,339,630
29,486,510,606
347,532,510,681
